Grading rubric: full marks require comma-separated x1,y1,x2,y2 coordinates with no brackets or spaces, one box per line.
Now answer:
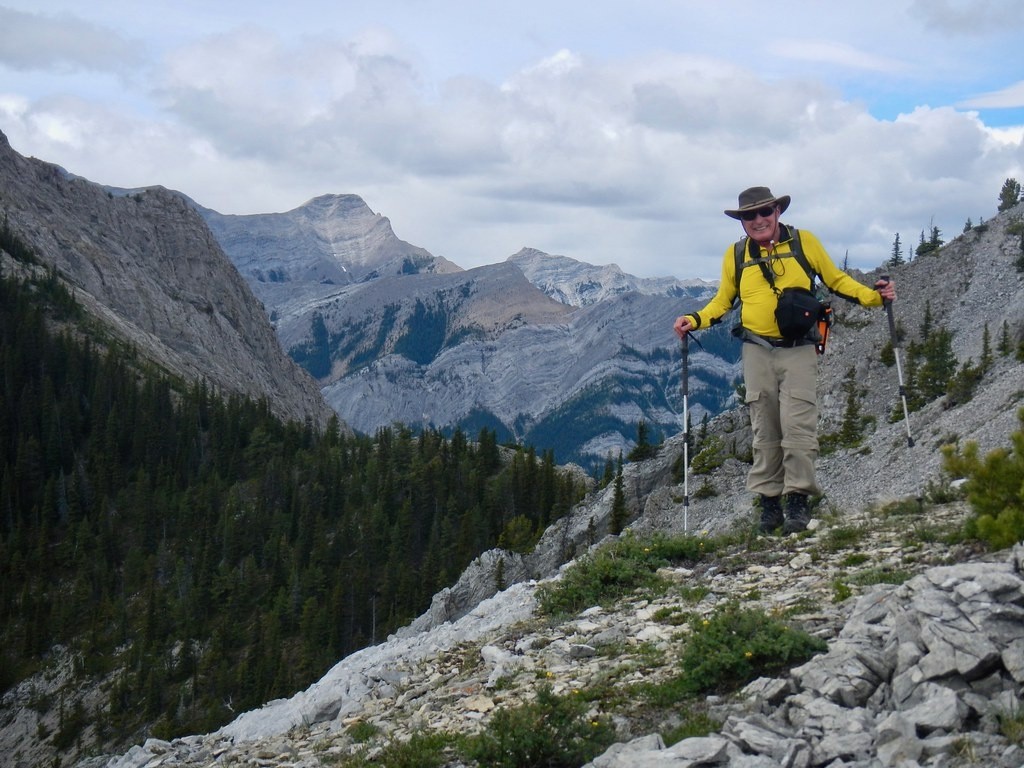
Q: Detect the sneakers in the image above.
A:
758,495,785,534
782,492,809,535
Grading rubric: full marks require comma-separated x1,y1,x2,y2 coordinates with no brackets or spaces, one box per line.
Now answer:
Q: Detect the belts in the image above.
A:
744,334,817,347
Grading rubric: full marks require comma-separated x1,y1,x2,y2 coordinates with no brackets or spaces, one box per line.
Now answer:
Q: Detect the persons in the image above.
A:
673,187,897,535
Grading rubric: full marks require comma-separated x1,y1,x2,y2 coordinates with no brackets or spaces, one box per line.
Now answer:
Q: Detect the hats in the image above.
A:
724,187,791,220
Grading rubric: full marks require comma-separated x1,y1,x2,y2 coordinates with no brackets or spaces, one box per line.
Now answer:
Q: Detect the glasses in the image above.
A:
740,205,776,221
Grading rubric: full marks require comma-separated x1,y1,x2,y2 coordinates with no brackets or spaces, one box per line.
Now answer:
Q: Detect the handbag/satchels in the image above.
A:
774,286,820,339
